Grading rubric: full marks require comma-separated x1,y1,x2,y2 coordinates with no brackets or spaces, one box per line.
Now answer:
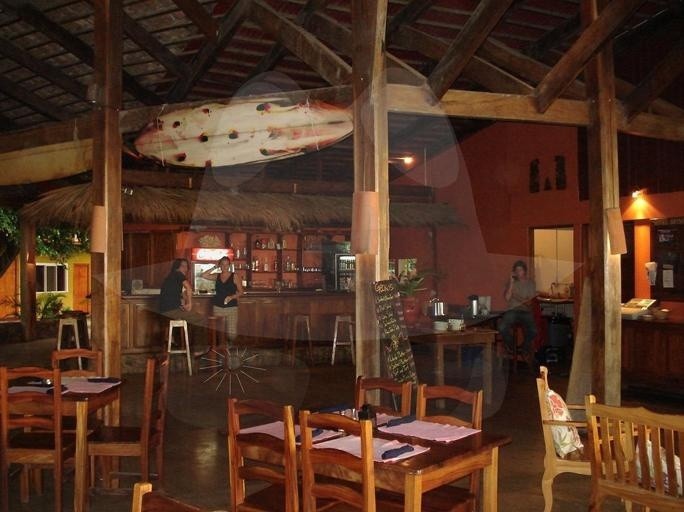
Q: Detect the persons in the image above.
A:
495,260,537,359
158,258,210,358
201,256,243,345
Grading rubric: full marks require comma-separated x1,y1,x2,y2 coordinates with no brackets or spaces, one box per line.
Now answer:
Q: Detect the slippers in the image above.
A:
194,346,212,361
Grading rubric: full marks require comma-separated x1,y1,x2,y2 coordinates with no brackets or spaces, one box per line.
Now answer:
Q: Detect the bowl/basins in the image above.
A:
432,320,448,331
447,319,464,331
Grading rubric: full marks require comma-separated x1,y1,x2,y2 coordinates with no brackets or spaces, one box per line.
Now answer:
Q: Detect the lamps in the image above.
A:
603,206,628,256
90,205,124,254
350,188,379,256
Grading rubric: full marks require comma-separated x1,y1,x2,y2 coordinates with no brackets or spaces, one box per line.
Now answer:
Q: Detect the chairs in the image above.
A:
536,365,632,512
584,395,684,512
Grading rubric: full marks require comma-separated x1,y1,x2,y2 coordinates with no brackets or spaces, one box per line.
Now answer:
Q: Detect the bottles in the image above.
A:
303,266,322,273
338,407,357,433
285,255,299,270
281,279,295,289
339,260,356,271
251,235,279,273
234,247,249,270
241,273,248,288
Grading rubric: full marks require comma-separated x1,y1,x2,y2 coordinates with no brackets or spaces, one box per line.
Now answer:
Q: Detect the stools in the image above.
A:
510,323,525,375
330,315,356,366
164,320,192,377
288,313,315,369
57,312,84,371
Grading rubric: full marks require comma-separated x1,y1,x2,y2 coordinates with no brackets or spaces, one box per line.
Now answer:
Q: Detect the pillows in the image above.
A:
546,390,584,459
636,438,683,497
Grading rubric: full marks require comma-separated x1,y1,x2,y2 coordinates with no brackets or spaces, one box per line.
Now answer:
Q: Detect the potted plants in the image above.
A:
392,259,446,329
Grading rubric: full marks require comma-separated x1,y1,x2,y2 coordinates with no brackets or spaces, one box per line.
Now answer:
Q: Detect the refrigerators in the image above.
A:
189,248,234,293
332,242,355,292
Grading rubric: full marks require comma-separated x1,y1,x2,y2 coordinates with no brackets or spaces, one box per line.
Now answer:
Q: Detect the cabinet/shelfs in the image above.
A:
125,222,351,291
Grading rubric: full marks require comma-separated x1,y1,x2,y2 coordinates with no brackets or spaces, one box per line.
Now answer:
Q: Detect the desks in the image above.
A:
423,311,505,367
405,328,498,408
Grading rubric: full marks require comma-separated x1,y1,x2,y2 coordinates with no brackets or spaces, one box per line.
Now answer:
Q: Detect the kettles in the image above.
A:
428,297,446,320
466,294,479,318
